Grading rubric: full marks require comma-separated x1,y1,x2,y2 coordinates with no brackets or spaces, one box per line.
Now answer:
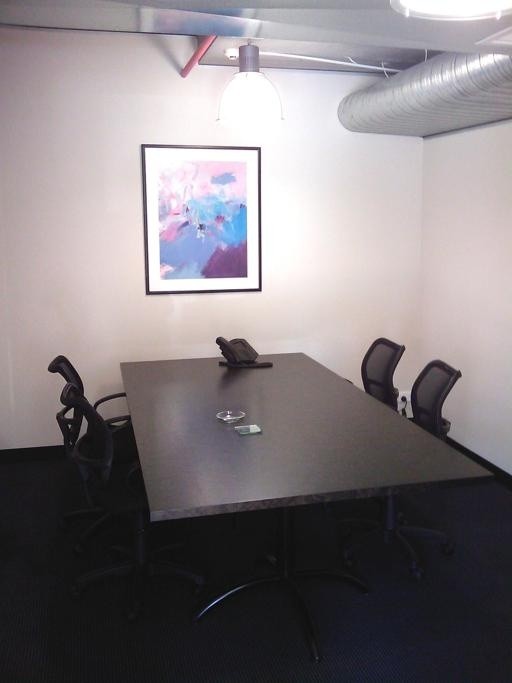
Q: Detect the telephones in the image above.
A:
216,337,259,364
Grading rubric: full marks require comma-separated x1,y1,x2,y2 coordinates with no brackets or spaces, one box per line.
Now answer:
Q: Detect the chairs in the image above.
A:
61,381,207,623
48,354,133,557
336,361,462,582
360,337,407,418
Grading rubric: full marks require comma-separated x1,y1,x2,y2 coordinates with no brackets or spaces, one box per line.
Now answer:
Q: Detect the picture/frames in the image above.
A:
140,142,263,294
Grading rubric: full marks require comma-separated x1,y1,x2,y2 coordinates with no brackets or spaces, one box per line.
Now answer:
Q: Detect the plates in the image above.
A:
216,410,245,423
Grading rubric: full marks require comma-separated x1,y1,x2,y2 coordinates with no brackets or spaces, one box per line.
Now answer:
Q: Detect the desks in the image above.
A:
120,353,494,664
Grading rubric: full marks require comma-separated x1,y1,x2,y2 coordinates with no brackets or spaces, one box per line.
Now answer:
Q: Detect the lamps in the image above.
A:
216,36,283,127
